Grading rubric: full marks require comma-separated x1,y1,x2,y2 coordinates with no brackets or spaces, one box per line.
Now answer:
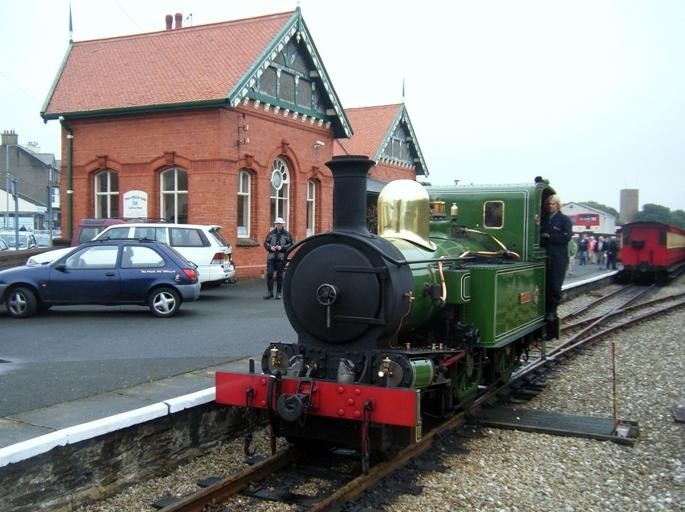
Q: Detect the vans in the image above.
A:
28,223,238,294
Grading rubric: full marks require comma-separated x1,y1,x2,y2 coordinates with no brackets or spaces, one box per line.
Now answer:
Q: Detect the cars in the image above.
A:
0,227,62,254
0,239,202,318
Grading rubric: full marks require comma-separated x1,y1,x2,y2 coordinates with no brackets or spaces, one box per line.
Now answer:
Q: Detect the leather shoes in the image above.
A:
263,291,273,299
276,292,281,299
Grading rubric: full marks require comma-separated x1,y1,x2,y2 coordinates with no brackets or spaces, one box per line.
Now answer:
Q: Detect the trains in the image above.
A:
213,176,557,469
616,222,685,287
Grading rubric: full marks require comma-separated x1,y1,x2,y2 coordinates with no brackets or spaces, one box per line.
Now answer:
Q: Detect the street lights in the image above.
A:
11,176,22,251
46,184,55,242
6,142,37,226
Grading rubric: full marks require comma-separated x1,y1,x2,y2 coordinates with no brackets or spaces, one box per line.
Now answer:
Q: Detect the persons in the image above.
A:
263,217,294,300
579,233,621,272
540,193,572,341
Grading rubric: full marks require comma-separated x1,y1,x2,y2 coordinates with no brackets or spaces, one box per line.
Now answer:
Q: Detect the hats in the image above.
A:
273,218,285,223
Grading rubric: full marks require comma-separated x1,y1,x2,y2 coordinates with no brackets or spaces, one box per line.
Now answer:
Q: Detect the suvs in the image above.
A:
69,216,154,248
